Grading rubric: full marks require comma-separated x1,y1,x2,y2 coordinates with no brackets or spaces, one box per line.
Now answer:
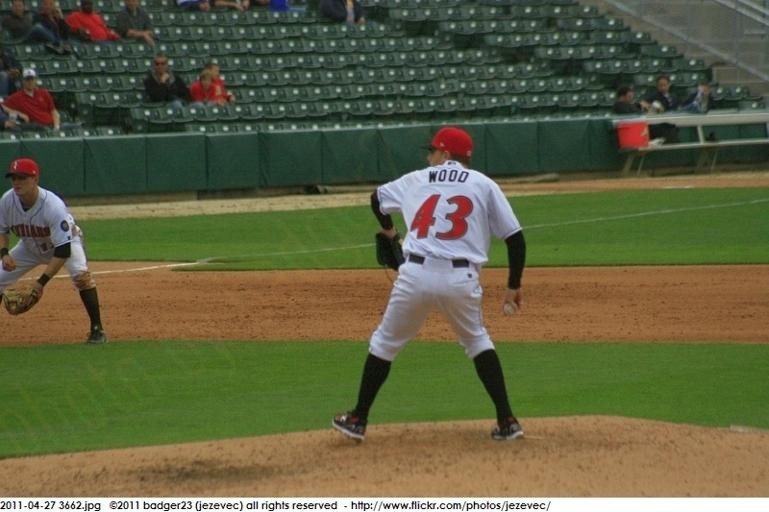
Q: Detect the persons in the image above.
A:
637,75,680,143
0,159,106,343
324,0,365,25
682,83,717,142
613,88,638,115
0,1,250,133
332,126,527,445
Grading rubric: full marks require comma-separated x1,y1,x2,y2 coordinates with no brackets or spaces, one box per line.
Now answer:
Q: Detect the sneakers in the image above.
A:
86,323,108,344
331,411,368,441
491,416,524,441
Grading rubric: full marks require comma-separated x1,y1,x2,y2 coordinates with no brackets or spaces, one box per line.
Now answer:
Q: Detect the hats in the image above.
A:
422,127,473,161
5,158,40,178
20,67,37,81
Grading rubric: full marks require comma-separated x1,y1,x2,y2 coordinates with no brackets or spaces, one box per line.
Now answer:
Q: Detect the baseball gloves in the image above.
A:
375,231,405,270
3,281,42,314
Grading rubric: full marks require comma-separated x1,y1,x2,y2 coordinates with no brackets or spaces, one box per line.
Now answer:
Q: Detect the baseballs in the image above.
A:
503,302,517,315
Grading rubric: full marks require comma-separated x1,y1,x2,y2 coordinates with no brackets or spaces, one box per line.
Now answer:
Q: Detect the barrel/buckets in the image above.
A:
616,118,651,149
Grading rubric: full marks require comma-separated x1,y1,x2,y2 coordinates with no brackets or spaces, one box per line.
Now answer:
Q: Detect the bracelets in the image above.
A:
37,274,50,287
0,247,9,258
